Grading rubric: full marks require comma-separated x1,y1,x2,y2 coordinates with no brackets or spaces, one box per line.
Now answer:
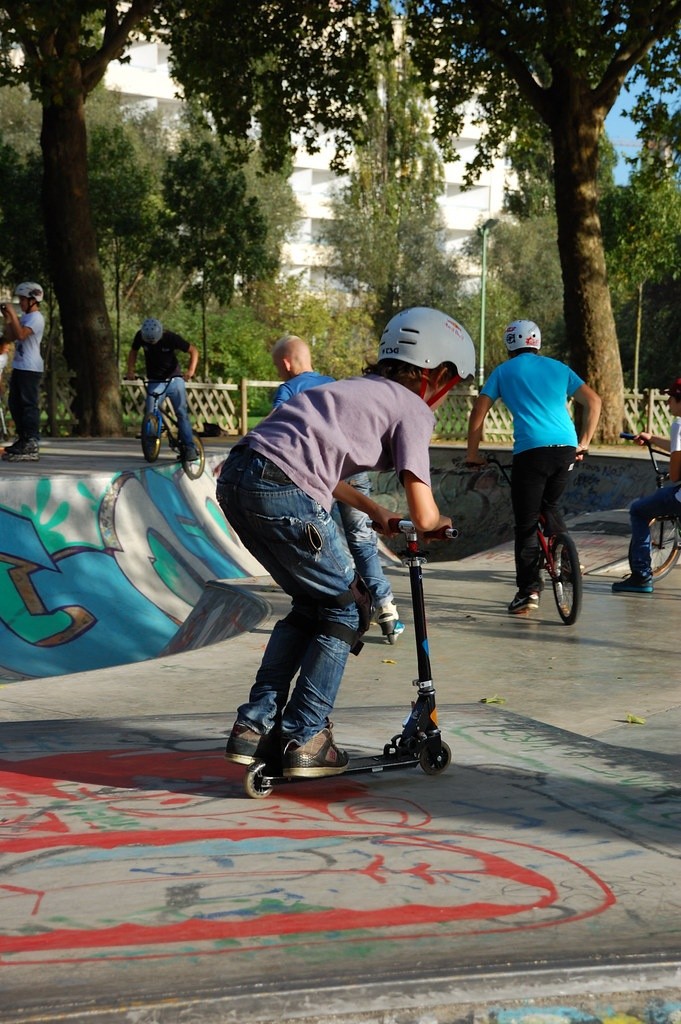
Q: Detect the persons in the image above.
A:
0,282,48,461
126,319,198,462
612,379,681,591
465,320,602,613
217,308,477,777
212,335,405,644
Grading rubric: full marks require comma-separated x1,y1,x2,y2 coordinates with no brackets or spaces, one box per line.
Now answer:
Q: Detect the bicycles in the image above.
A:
461,448,588,624
619,433,681,584
123,375,204,481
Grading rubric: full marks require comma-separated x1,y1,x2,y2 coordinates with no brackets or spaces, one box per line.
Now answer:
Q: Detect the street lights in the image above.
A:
476,218,500,441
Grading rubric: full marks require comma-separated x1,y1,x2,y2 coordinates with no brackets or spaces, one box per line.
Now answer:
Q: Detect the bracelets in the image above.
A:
579,444,588,449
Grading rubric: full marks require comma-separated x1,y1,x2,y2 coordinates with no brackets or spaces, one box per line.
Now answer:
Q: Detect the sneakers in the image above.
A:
224,721,278,765
612,570,653,592
279,716,350,778
508,592,540,613
380,620,405,645
3,435,40,461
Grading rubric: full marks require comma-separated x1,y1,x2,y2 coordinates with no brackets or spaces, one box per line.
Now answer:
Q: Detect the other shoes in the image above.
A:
185,449,196,460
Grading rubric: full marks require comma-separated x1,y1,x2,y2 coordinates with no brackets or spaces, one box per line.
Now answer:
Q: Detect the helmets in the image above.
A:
15,282,44,302
378,307,476,379
141,319,163,344
504,320,541,351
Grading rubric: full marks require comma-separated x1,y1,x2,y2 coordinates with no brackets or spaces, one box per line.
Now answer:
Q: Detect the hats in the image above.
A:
664,378,681,396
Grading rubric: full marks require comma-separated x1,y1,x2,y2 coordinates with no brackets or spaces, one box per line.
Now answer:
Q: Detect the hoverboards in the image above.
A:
246,515,457,800
